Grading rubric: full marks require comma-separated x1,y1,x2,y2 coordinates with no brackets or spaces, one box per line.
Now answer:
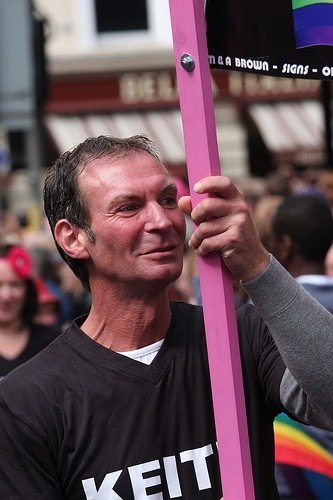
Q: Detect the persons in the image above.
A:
0,244,66,381
250,191,333,500
0,130,333,500
0,163,333,330
